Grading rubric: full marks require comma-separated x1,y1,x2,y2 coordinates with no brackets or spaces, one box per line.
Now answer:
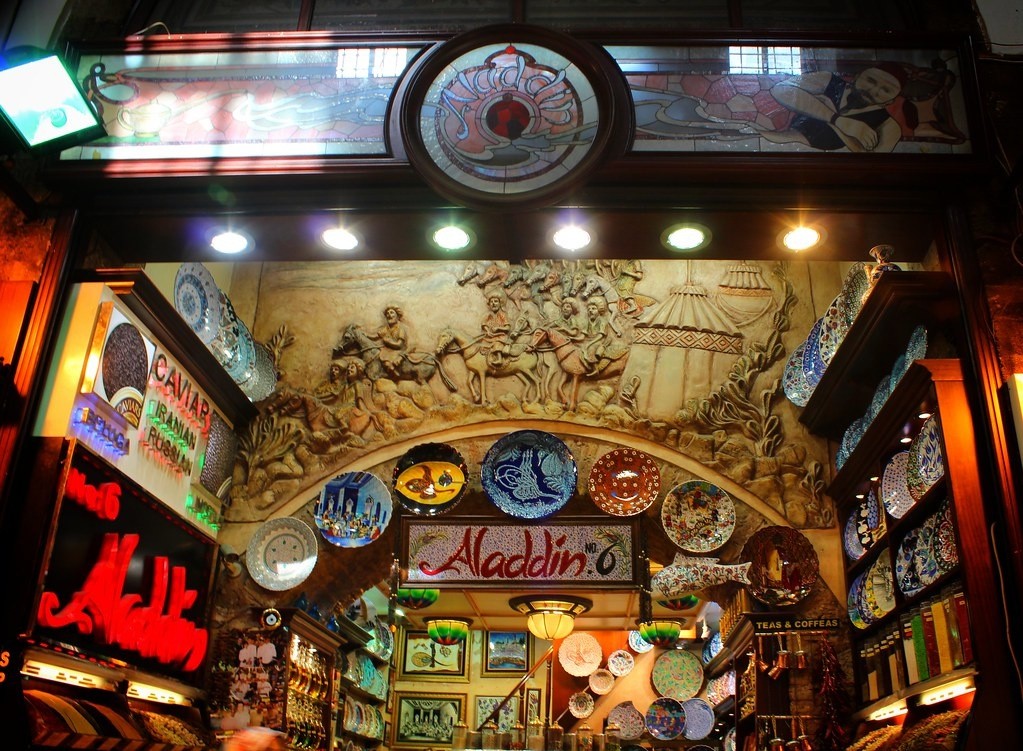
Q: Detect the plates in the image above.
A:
662,479,737,553
587,448,660,517
245,517,318,592
781,260,957,631
341,593,394,739
480,430,578,519
313,471,394,549
173,262,277,403
738,525,818,606
391,442,469,517
558,630,736,751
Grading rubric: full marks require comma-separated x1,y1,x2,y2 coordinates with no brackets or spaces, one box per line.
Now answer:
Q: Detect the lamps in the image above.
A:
0,45,108,160
397,588,440,610
509,595,593,640
659,595,700,611
422,616,473,645
635,618,688,647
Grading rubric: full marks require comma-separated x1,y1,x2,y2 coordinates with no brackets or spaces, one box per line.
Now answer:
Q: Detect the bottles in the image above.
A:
284,637,329,751
718,591,754,717
860,245,903,307
452,718,621,751
294,591,340,632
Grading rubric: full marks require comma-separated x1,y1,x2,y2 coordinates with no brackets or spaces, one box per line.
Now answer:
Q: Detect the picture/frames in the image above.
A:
480,630,535,678
386,686,394,714
391,692,468,751
474,694,519,731
383,721,391,747
403,630,466,676
390,654,396,669
525,689,543,749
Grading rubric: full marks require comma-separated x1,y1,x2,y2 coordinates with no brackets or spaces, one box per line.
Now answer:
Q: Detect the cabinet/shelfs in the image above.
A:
251,607,391,751
798,270,1023,751
704,615,791,751
